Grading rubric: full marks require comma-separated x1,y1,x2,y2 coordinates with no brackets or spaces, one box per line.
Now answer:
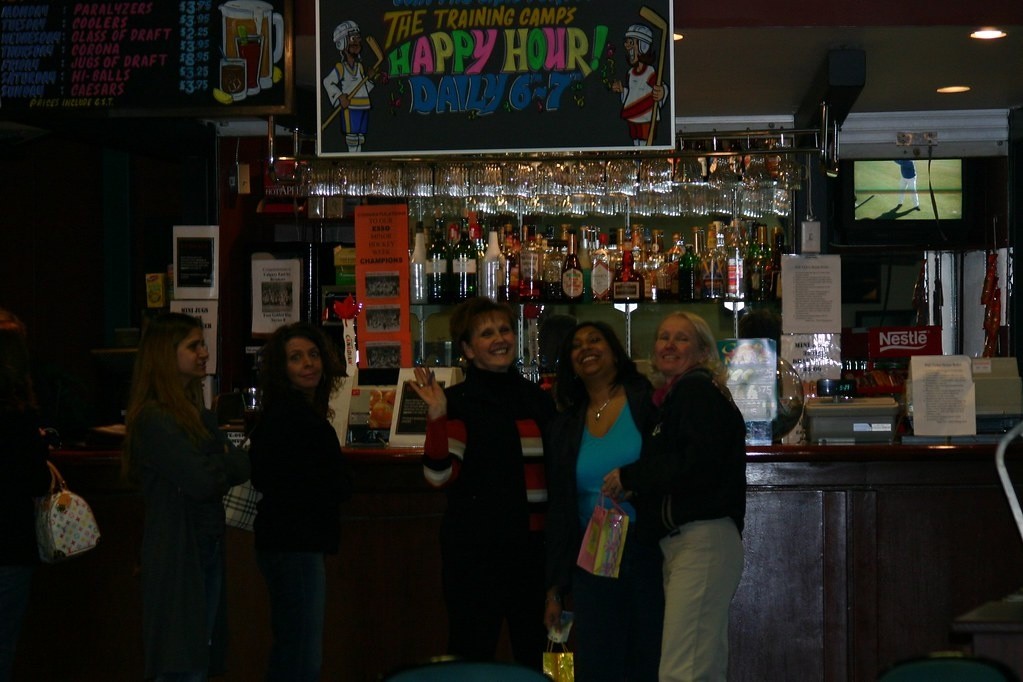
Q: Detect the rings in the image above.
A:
419,383,425,388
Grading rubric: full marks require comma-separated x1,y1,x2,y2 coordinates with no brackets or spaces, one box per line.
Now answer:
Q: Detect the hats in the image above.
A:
0,309,27,337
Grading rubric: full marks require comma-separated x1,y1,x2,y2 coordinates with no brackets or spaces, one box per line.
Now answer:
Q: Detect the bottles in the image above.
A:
410,207,786,304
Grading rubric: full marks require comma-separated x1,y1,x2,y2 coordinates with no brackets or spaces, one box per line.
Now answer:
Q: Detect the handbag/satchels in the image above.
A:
32,459,102,566
542,639,575,682
221,423,265,533
576,490,630,578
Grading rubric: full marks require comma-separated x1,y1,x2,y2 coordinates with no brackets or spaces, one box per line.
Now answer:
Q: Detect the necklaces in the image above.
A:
591,390,615,421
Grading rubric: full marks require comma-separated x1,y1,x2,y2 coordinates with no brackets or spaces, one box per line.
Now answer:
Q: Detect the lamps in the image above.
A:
971,26,1006,39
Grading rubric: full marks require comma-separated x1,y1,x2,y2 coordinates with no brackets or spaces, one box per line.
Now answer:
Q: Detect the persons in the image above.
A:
894,160,921,211
544,320,664,682
409,297,560,674
243,323,350,682
123,312,251,682
602,312,747,682
740,273,782,349
0,310,53,682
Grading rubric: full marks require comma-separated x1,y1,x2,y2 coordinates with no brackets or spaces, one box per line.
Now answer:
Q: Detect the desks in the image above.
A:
953,601,1023,682
0,434,1023,682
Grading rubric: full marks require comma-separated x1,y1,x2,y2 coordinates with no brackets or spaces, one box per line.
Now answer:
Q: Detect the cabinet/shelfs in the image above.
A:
270,129,821,407
